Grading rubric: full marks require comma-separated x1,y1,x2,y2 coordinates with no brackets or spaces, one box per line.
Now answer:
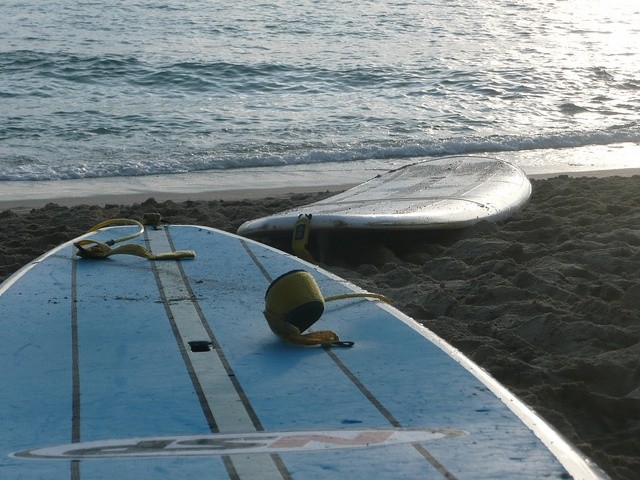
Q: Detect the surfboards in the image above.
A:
237,155,533,234
0,223,609,480
